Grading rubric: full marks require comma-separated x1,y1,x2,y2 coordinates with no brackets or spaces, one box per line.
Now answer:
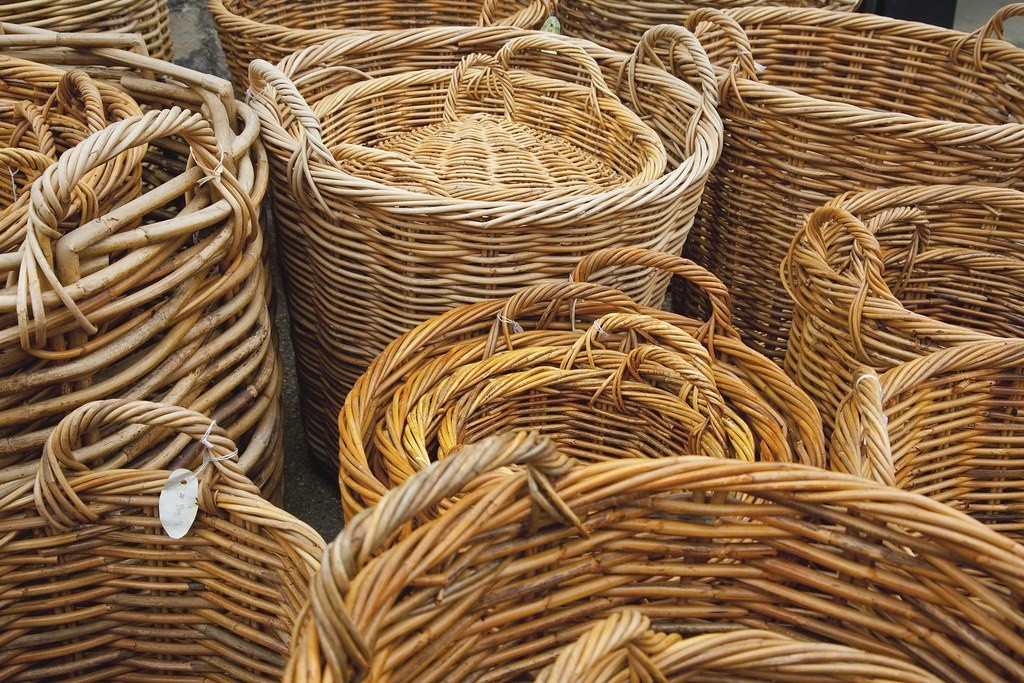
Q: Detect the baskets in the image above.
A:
2,3,1023,682
